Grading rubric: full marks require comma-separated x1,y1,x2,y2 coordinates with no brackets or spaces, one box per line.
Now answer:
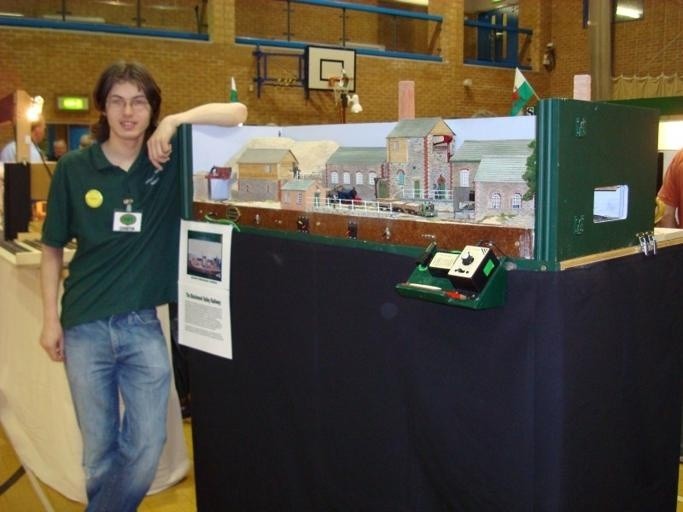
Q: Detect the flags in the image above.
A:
229,75,243,128
509,65,535,115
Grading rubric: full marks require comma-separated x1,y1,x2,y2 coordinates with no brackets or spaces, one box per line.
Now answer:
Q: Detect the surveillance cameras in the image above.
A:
546,42,554,50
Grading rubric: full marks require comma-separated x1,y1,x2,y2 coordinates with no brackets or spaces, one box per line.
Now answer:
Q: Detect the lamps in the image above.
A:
347,94,363,114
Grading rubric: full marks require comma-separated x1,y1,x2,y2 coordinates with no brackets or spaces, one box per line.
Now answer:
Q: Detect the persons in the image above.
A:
328,184,357,205
459,197,469,210
254,213,261,225
46,137,68,161
424,201,434,211
78,135,96,148
437,173,446,200
296,215,310,233
38,59,249,512
0,114,47,162
655,147,682,227
345,220,359,240
290,161,300,179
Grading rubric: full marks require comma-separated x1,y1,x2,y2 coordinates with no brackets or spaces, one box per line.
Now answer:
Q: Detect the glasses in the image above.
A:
106,95,150,112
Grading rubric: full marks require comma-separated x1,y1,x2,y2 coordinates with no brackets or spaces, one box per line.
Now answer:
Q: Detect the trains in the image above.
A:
379,201,435,218
